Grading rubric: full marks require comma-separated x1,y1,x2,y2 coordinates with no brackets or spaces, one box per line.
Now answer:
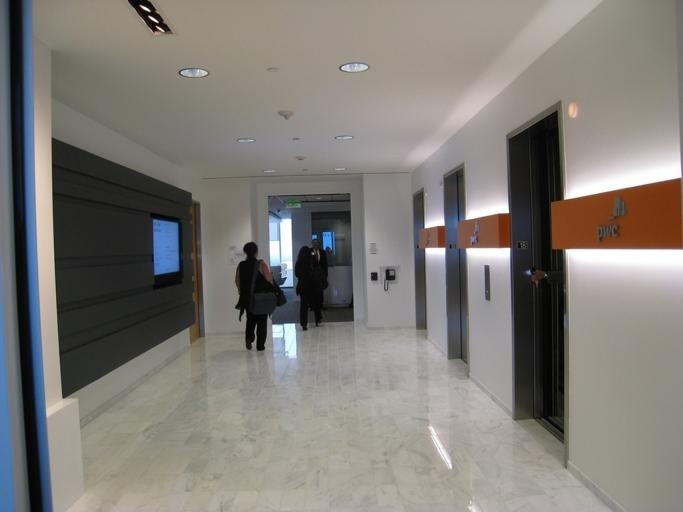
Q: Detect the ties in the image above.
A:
315,250,318,265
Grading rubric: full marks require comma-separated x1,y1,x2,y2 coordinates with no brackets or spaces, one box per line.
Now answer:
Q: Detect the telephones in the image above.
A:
386,269,395,280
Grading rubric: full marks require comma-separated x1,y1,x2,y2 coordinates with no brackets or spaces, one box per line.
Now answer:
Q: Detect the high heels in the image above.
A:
302,326,308,331
315,322,321,327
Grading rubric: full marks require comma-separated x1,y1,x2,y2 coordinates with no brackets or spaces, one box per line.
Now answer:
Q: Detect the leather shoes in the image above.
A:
246,338,251,350
256,345,265,351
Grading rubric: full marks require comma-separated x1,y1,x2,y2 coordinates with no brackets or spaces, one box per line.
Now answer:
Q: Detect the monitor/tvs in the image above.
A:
152,216,181,277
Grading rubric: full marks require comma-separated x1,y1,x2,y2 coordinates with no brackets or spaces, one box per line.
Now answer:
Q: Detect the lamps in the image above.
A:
128,0,174,35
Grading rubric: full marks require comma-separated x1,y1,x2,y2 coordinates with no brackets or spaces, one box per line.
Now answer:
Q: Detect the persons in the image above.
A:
235,242,274,351
295,239,328,331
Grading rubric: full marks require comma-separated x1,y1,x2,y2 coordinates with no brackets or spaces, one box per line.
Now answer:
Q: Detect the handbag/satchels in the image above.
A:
247,276,288,315
